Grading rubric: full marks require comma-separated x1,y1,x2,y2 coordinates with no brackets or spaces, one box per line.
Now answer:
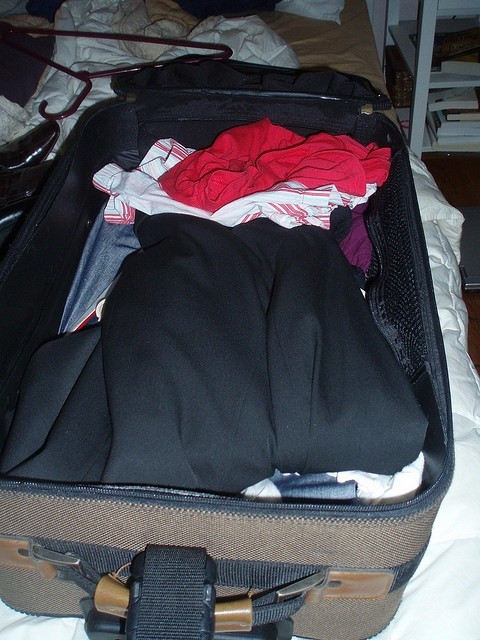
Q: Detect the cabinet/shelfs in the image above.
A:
386,0,480,158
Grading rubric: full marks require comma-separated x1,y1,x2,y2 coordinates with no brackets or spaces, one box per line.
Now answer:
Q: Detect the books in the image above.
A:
388,19,480,152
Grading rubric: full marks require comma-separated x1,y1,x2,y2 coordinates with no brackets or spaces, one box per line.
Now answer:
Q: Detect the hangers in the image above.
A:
4,26,233,120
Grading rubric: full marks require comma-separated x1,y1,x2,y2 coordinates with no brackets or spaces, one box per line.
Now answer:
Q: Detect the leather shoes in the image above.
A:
0,117,61,170
0,159,54,234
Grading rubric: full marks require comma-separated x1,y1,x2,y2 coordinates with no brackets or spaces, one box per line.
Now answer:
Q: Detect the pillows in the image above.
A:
275,0,346,24
